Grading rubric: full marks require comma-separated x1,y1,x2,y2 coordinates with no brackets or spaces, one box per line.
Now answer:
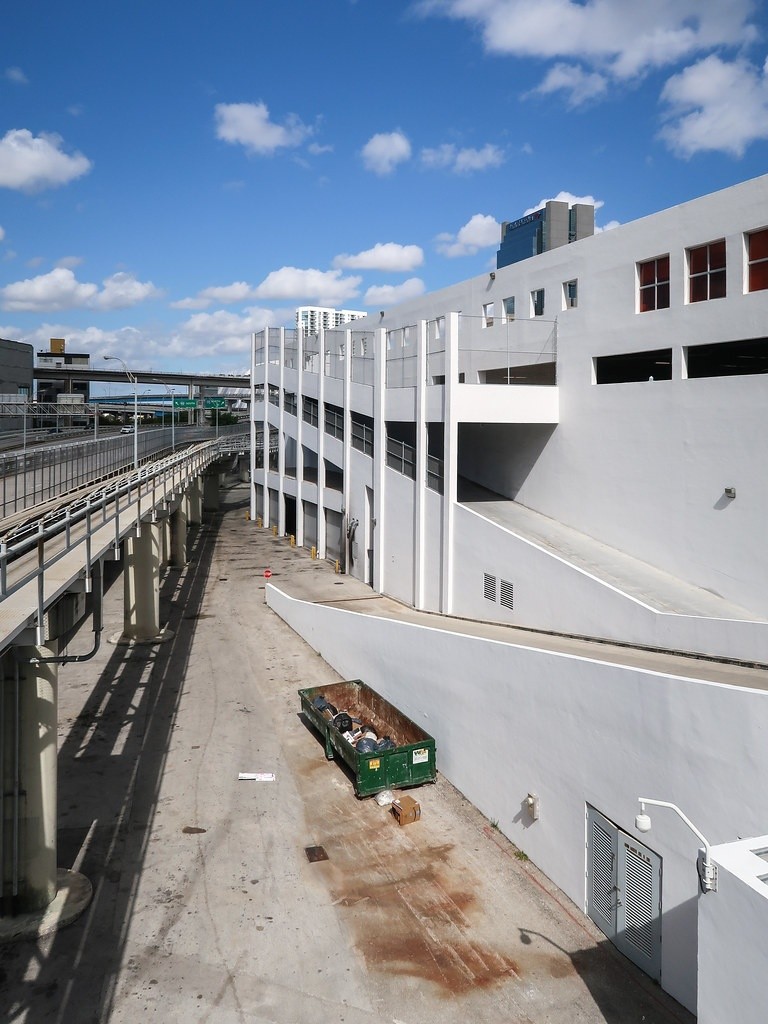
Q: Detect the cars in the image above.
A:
121,425,134,434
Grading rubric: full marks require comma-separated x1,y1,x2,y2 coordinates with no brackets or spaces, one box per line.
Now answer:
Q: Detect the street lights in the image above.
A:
23,389,47,452
162,389,175,429
153,379,175,453
197,392,218,439
140,389,152,425
103,355,138,468
124,393,135,425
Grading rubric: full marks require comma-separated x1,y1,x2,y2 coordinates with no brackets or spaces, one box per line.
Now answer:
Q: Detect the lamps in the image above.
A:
490,272,495,280
380,310,384,317
724,486,737,499
633,792,717,885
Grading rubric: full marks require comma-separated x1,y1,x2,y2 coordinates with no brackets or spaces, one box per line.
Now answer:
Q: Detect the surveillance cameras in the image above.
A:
635,816,651,833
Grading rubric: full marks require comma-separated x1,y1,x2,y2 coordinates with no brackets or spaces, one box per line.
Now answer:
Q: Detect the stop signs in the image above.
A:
264,569,272,579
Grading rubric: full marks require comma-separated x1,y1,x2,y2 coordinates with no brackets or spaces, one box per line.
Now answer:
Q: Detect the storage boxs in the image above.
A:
389,794,421,825
297,678,438,797
